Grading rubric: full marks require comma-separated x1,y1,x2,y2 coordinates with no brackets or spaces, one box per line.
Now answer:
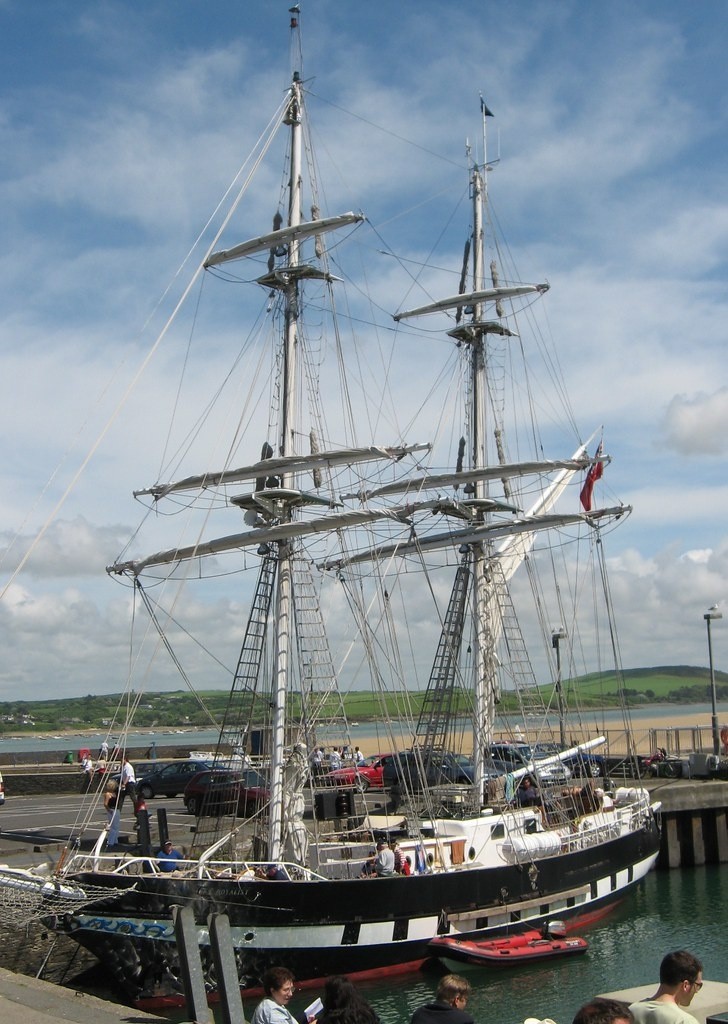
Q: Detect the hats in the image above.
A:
377,839,387,844
164,839,172,845
266,864,277,871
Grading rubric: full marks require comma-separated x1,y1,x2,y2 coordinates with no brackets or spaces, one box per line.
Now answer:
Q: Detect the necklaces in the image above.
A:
266,864,287,881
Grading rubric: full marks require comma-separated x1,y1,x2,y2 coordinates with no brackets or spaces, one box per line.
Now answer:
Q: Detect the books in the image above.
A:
304,997,323,1021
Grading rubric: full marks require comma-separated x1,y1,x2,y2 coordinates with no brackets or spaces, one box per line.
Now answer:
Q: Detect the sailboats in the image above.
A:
51,71,668,1009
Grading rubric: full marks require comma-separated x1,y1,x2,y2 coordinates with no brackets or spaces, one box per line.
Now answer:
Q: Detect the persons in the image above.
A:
628,951,703,1024
105,780,121,849
308,745,364,788
136,792,147,816
519,777,536,808
251,967,318,1024
116,756,139,816
411,974,474,1024
155,839,190,871
570,1000,635,1024
316,975,380,1024
62,741,119,787
360,837,407,878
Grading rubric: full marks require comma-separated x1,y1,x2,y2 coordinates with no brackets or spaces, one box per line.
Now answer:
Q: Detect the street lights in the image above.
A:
703,612,723,755
551,634,569,751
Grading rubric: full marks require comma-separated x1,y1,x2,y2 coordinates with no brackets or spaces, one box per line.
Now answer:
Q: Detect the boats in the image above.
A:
422,923,587,967
8,721,359,741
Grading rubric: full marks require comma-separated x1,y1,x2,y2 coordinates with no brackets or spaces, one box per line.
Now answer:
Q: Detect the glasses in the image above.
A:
683,979,704,991
279,987,296,993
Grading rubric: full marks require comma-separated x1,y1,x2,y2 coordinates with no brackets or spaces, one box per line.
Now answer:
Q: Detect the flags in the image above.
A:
579,434,603,512
480,98,494,117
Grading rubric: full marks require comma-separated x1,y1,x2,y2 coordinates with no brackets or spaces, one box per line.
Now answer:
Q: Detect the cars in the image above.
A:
533,742,605,778
382,748,507,797
111,761,170,791
183,769,270,820
481,741,570,786
136,759,225,801
328,754,396,793
